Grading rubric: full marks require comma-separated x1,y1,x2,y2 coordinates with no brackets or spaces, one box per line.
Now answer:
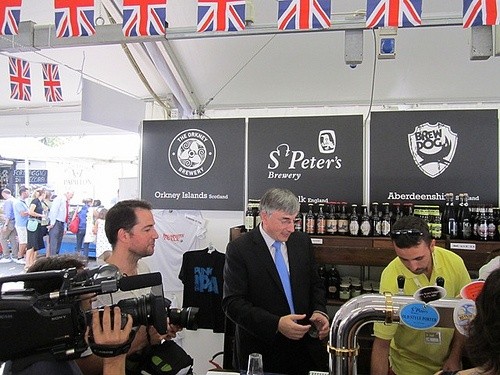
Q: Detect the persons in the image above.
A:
0,186,112,266
85,199,183,341
222,186,330,375
371,215,473,375
455,268,500,375
3,252,133,375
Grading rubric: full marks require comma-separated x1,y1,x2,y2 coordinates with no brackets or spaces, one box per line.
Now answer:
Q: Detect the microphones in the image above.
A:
38,271,162,301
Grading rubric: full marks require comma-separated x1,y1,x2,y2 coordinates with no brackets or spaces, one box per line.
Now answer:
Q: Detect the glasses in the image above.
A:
390,229,424,238
276,218,302,225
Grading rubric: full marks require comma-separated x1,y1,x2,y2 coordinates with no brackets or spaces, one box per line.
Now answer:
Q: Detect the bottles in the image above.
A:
320,267,340,300
247,353,264,375
245,193,500,242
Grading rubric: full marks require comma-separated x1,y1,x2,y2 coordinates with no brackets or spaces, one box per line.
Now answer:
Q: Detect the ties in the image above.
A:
66,201,68,223
272,241,297,323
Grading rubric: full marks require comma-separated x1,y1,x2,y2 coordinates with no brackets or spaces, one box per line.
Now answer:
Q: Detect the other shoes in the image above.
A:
0,257,30,271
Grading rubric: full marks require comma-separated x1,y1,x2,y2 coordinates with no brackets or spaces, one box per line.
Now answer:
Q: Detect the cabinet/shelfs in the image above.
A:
230,225,500,307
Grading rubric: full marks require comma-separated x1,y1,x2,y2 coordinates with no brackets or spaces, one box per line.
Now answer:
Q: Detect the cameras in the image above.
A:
168,307,200,330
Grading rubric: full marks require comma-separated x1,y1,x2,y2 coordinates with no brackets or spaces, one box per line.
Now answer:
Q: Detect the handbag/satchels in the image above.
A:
27,220,38,232
69,214,80,234
41,210,50,225
128,340,194,375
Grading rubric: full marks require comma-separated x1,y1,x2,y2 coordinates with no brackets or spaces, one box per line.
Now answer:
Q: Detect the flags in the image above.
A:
365,0,422,28
277,0,331,31
122,0,166,37
54,0,94,39
0,0,22,36
196,0,244,33
462,1,500,29
41,63,63,102
9,58,31,101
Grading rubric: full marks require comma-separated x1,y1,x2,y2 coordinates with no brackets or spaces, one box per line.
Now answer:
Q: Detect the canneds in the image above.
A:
340,278,380,300
